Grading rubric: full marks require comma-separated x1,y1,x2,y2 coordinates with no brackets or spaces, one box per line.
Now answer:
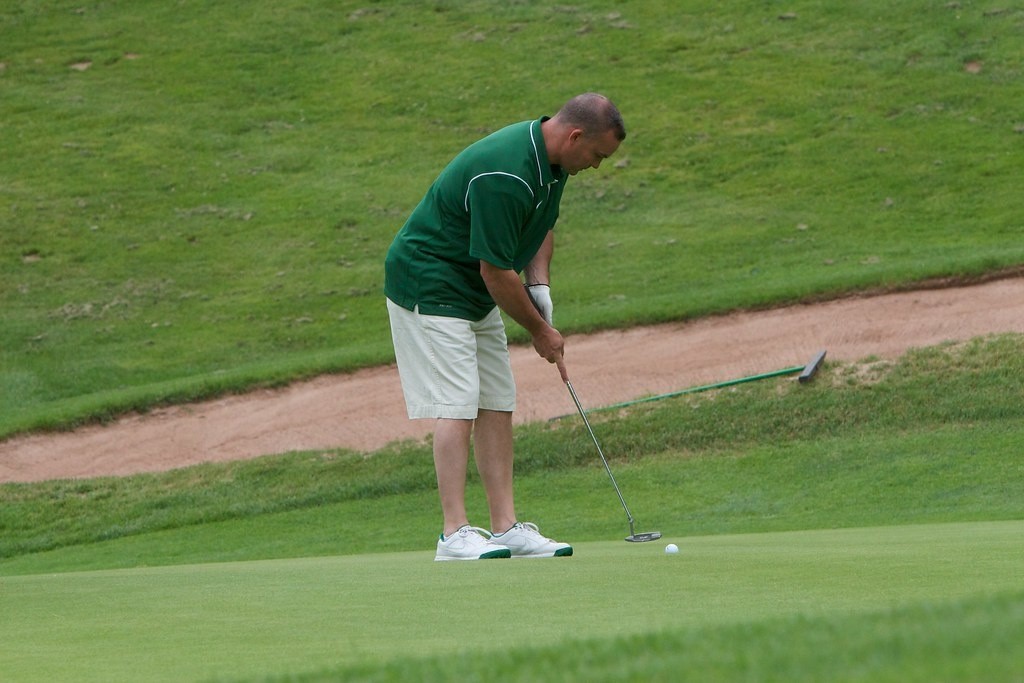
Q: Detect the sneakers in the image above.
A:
434,524,512,560
490,522,573,557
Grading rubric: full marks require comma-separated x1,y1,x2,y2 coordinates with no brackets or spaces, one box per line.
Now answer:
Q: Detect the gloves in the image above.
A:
527,284,554,328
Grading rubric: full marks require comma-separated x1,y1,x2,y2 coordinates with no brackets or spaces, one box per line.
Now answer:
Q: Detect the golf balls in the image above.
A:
664,543,680,554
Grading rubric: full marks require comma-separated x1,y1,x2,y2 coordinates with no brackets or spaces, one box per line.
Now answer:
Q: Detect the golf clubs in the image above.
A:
565,380,663,542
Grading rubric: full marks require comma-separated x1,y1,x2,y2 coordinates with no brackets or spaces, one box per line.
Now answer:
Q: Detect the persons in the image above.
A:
384,94,626,562
